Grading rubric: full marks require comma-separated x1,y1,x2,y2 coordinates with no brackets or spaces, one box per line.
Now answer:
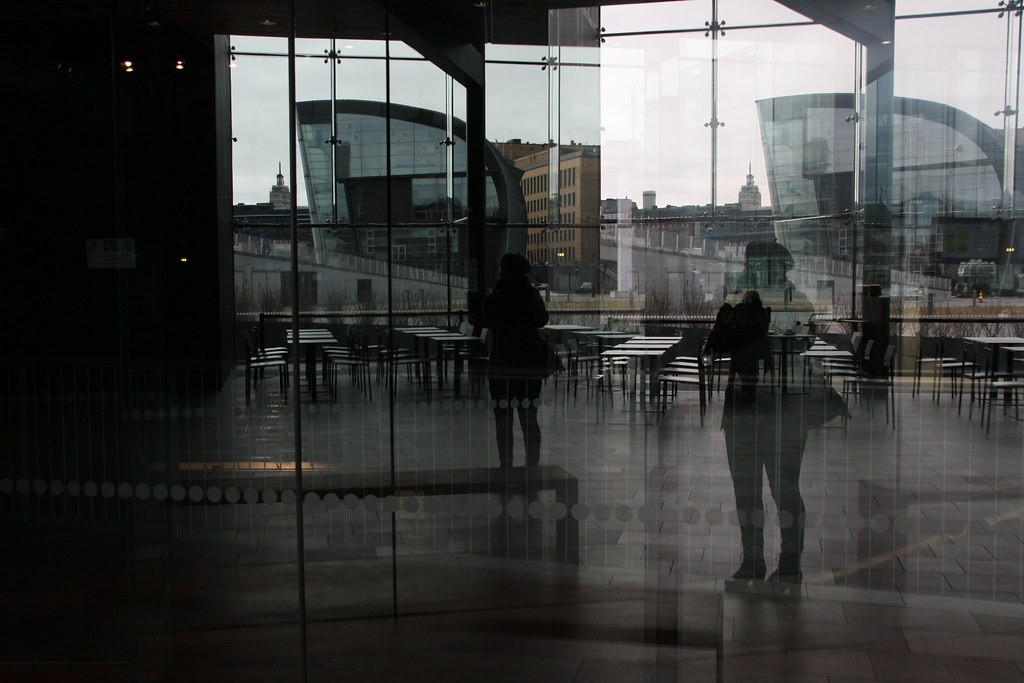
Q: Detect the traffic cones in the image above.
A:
977,291,984,303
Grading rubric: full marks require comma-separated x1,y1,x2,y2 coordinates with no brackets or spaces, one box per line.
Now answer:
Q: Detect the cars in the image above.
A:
580,282,592,293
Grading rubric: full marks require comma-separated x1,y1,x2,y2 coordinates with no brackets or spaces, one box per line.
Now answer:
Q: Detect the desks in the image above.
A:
957,336,1024,421
394,327,485,399
798,335,852,391
1000,347,1024,415
547,324,683,427
286,328,337,406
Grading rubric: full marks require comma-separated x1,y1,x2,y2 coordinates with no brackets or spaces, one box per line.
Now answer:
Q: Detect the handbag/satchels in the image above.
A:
804,365,845,427
530,336,561,380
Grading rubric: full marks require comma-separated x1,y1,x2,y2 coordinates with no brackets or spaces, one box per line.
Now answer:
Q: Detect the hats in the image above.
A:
745,240,794,272
500,251,530,274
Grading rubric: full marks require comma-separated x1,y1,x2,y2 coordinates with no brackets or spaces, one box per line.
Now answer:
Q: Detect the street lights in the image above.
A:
569,268,578,291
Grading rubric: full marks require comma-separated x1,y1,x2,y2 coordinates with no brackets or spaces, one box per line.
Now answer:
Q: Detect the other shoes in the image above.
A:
754,569,802,596
724,559,767,590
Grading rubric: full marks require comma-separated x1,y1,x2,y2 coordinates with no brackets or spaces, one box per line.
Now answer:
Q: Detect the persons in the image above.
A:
719,242,842,601
706,291,770,403
465,255,550,469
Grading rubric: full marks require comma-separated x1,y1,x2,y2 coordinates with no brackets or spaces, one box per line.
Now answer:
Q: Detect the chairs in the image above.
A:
658,336,722,427
460,321,493,392
320,326,373,401
912,336,1024,435
244,311,290,405
374,326,429,392
822,332,898,430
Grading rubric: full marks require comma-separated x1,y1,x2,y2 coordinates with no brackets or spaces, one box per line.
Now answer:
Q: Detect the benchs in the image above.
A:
155,463,580,568
